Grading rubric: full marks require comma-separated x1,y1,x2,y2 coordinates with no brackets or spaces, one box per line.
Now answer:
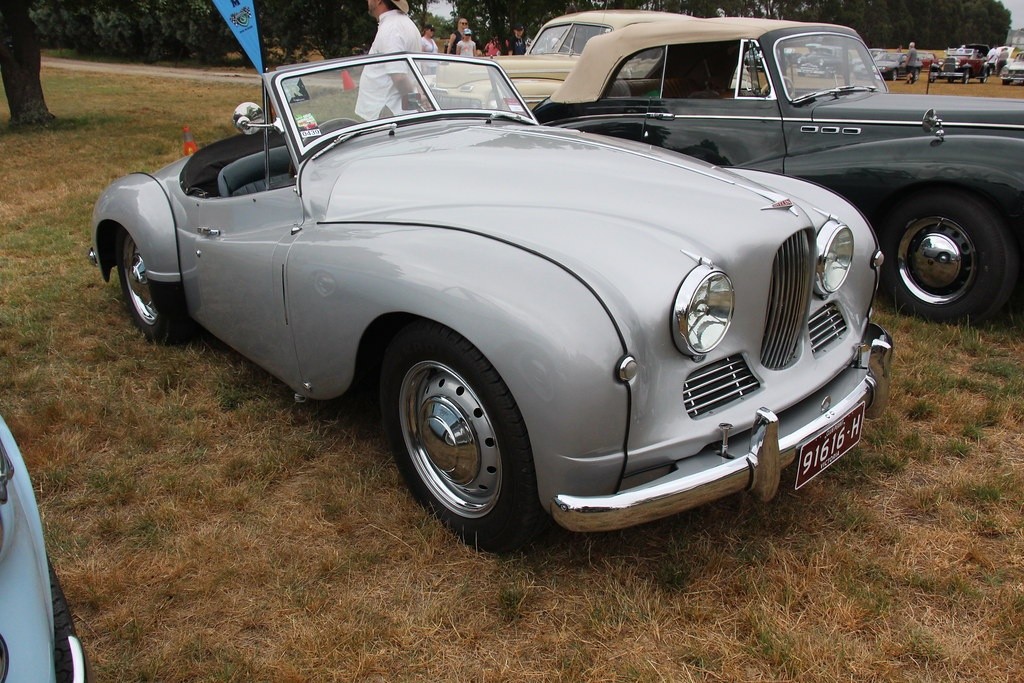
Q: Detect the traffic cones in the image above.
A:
181,123,199,155
342,68,359,92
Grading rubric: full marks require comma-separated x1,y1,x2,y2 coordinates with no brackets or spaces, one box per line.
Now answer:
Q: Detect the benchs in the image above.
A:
218,144,297,197
610,77,726,99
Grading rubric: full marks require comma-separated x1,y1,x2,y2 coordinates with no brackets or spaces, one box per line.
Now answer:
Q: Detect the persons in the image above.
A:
485,40,500,56
986,44,998,77
896,45,902,53
508,25,526,56
447,17,471,54
905,42,918,84
996,48,1009,77
456,28,477,56
355,0,422,121
421,24,439,53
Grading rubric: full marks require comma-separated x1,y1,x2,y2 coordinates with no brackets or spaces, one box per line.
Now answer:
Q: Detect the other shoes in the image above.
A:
911,79,916,84
905,81,909,84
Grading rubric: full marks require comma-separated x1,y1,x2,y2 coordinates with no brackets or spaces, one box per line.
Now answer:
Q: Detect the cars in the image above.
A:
741,41,1024,86
533,14,1024,323
428,9,755,114
88,49,892,548
0,413,87,682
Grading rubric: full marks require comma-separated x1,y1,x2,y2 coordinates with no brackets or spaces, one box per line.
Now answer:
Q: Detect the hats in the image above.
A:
464,29,471,34
391,0,410,15
425,24,436,31
565,5,577,14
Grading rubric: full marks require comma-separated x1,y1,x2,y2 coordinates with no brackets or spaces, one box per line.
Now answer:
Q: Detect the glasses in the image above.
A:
466,34,472,36
430,29,435,32
460,22,468,25
516,28,524,31
